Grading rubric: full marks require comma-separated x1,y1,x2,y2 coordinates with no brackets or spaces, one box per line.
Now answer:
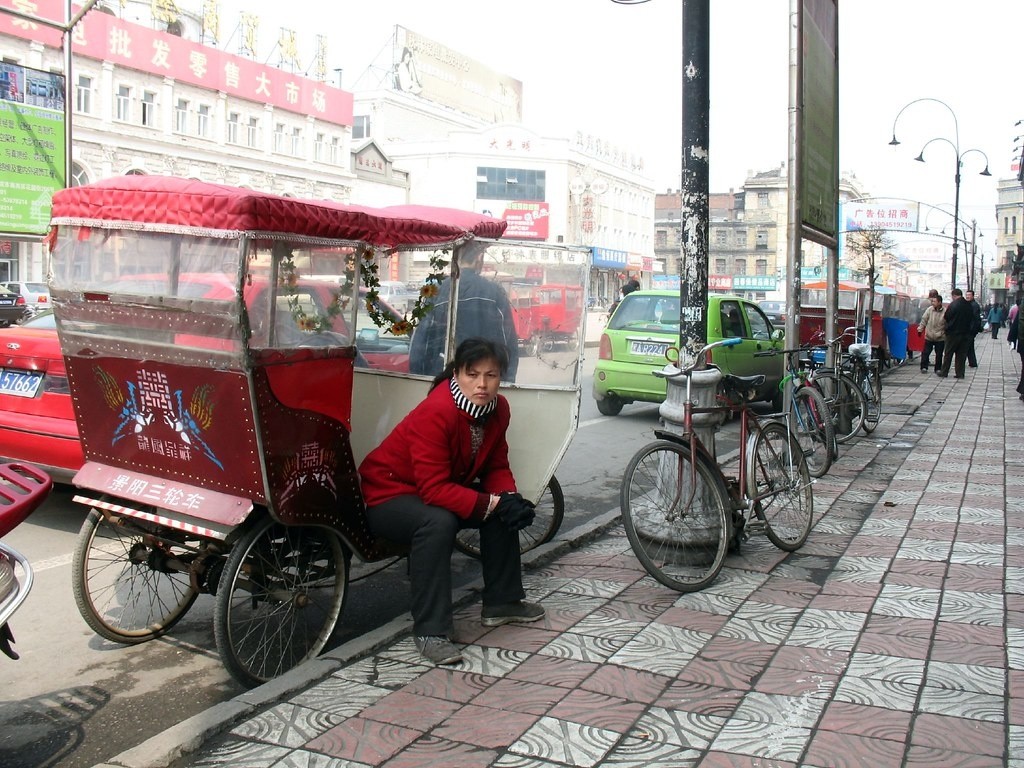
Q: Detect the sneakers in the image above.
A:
412,634,463,665
480,598,546,626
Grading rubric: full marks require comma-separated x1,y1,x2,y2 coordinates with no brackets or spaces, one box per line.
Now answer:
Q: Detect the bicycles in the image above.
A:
753,343,840,480
620,333,817,593
804,325,883,444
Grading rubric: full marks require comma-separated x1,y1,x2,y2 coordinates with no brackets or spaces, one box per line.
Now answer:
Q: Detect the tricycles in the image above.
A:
44,174,594,690
503,282,586,356
797,278,951,374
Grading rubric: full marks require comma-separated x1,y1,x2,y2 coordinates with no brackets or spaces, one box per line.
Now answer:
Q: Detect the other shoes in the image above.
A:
922,368,927,373
954,374,964,379
937,372,947,377
969,363,978,367
935,369,942,374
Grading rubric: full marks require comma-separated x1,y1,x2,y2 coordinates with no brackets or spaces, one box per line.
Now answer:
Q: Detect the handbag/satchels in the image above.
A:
983,322,990,332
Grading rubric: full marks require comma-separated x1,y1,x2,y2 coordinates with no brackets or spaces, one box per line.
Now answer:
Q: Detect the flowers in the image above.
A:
360,244,450,336
280,239,363,334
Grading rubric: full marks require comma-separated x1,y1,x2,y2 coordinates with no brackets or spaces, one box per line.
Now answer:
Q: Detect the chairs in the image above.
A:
660,310,679,324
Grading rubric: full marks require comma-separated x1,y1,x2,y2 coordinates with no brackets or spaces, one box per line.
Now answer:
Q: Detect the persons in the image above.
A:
410,242,520,381
937,289,974,378
358,339,546,664
981,303,1009,339
729,309,742,335
616,280,640,301
1008,299,1024,402
900,290,938,364
965,290,983,367
917,295,946,375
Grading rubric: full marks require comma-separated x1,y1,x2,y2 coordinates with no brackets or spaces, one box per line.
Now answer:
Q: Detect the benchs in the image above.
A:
54,301,359,499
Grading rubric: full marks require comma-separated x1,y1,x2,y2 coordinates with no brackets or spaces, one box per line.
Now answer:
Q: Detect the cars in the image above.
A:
291,276,439,320
0,271,412,493
0,284,28,328
751,300,786,332
593,287,786,427
0,280,52,325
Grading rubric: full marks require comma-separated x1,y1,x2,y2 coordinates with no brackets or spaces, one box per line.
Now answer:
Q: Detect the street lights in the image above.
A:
924,203,985,291
888,98,992,291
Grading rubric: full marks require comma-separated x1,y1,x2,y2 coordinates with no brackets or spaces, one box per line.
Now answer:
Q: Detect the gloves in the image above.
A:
483,492,538,531
